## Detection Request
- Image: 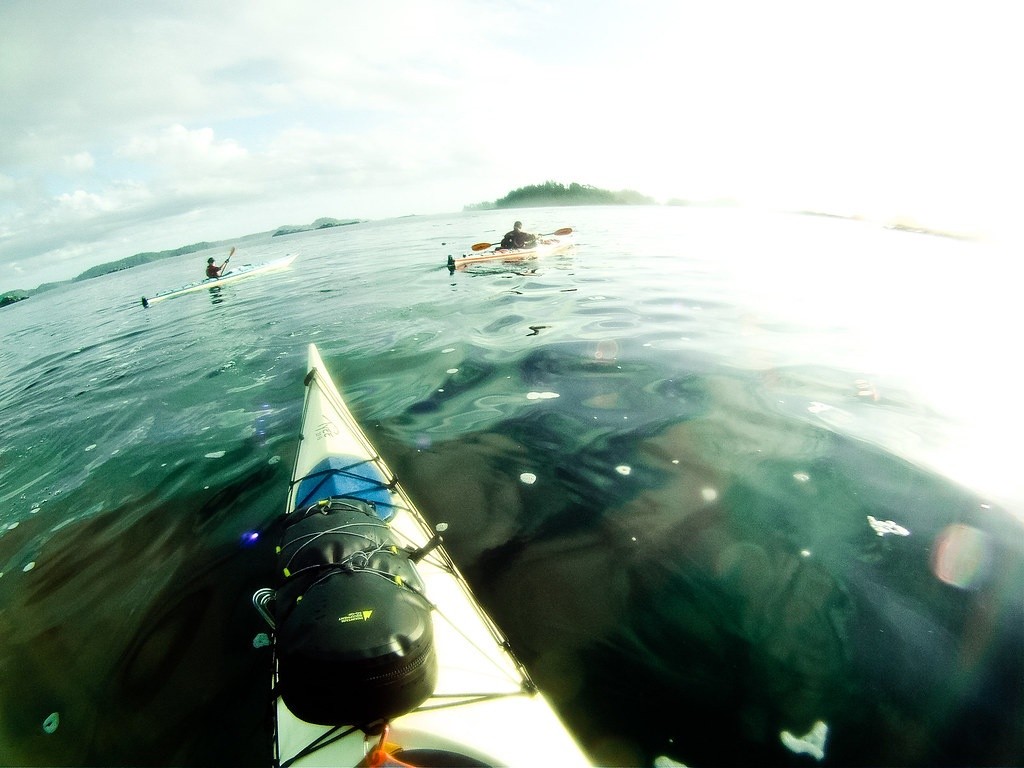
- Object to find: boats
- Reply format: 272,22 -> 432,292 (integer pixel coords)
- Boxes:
251,340 -> 604,768
140,251 -> 303,307
445,232 -> 570,272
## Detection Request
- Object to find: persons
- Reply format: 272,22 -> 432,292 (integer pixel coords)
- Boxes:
206,257 -> 228,278
502,222 -> 540,249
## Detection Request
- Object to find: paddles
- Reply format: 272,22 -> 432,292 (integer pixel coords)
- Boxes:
220,246 -> 235,276
471,227 -> 572,251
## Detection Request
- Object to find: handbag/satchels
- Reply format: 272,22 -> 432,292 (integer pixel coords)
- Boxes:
269,494 -> 437,728
